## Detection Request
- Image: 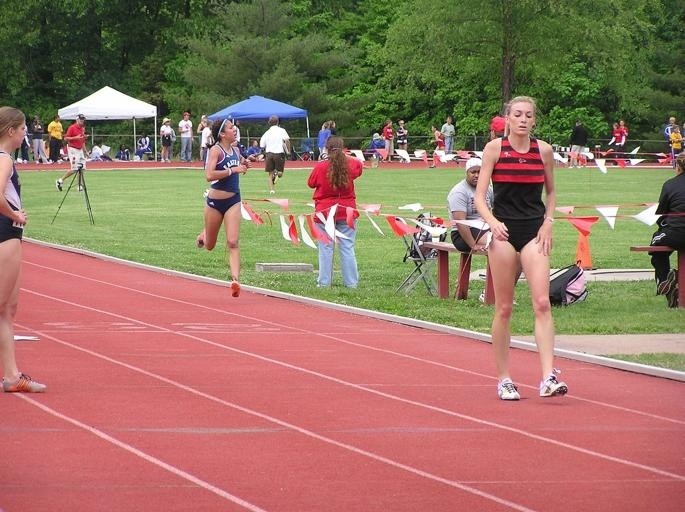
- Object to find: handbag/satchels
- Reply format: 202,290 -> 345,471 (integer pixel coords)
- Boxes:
162,135 -> 171,146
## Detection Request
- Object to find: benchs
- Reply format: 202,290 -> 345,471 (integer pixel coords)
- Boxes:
628,237 -> 685,316
364,152 -> 400,162
141,150 -> 153,160
421,236 -> 521,306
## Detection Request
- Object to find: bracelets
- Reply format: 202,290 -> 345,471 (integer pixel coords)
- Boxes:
543,213 -> 554,222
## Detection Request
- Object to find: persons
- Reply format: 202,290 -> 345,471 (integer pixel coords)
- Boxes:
21,115 -> 64,164
55,115 -> 90,192
135,131 -> 153,162
608,121 -> 629,165
159,115 -> 213,163
430,116 -> 455,168
448,158 -> 522,305
318,121 -> 337,160
233,121 -> 265,162
569,121 -> 588,167
370,120 -> 408,162
0,107 -> 45,393
307,136 -> 363,289
664,116 -> 682,160
197,120 -> 251,297
490,112 -> 506,140
475,96 -> 569,401
648,152 -> 684,308
260,116 -> 291,194
91,140 -> 129,161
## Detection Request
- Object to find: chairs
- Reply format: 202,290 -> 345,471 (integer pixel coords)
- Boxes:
387,213 -> 446,297
292,139 -> 315,161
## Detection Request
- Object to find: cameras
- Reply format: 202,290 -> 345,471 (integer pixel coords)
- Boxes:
75,162 -> 84,169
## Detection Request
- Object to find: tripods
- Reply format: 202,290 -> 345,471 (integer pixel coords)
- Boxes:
50,169 -> 95,225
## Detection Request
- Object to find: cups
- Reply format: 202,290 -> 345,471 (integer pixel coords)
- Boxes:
432,235 -> 440,242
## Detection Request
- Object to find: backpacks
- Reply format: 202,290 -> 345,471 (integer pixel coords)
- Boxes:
404,212 -> 447,261
550,260 -> 588,309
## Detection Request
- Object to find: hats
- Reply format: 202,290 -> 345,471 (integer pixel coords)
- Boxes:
491,116 -> 505,133
162,117 -> 171,124
465,158 -> 484,173
185,112 -> 190,117
77,113 -> 86,120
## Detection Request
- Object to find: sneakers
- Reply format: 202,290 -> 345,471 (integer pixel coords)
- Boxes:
56,179 -> 64,191
196,233 -> 204,248
230,280 -> 241,297
657,269 -> 678,295
539,374 -> 568,396
666,286 -> 678,308
497,378 -> 521,400
3,372 -> 46,393
